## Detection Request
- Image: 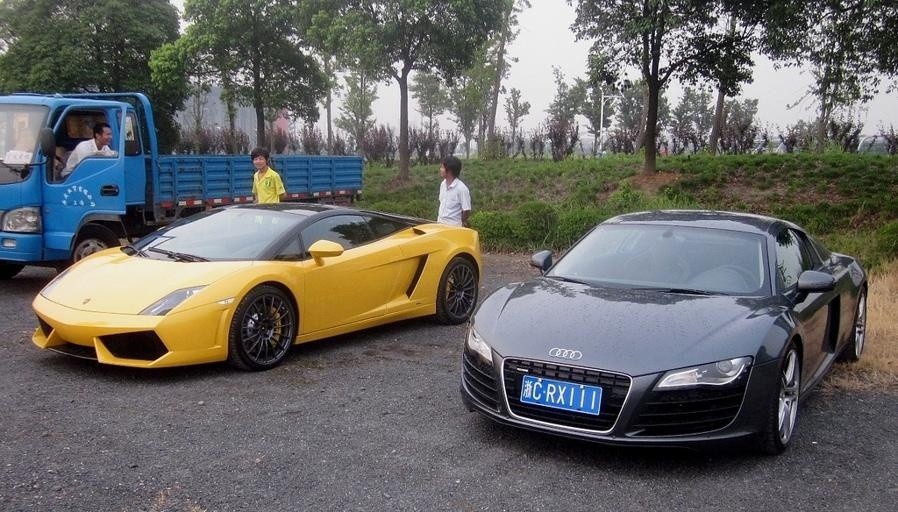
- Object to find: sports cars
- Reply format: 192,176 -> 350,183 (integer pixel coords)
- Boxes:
460,205 -> 870,461
30,201 -> 485,377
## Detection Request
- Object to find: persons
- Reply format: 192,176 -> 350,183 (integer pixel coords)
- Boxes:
436,156 -> 471,228
251,146 -> 286,203
60,123 -> 120,181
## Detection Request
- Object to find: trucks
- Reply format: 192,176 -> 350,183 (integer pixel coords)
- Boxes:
1,91 -> 366,286
